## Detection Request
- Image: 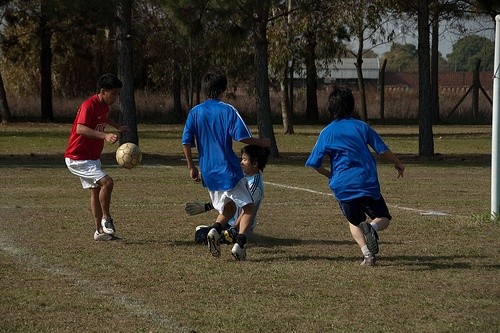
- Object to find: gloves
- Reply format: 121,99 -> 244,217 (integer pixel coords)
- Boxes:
185,200 -> 211,216
224,227 -> 236,242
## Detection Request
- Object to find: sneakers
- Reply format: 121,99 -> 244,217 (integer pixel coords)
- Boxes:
360,255 -> 376,267
207,228 -> 221,258
231,242 -> 248,261
359,221 -> 379,254
94,230 -> 114,241
101,217 -> 116,235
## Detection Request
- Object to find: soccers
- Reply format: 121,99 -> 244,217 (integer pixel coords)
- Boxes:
116,143 -> 142,169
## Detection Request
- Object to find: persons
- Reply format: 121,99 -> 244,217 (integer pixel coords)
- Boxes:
181,69 -> 271,262
185,143 -> 271,244
64,73 -> 131,240
304,85 -> 406,267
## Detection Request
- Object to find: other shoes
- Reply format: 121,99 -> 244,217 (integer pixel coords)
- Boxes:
196,225 -> 209,232
